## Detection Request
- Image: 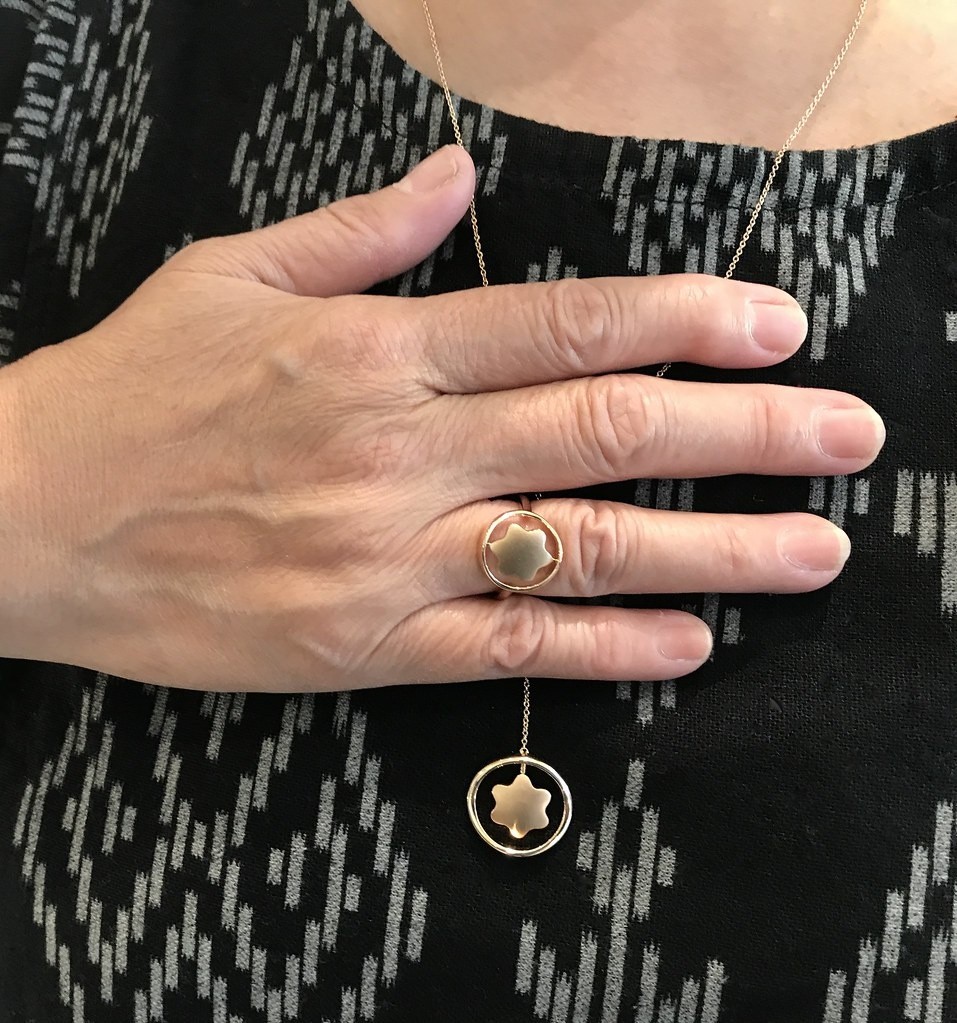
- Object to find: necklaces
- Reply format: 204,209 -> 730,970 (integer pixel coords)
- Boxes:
420,4 -> 869,858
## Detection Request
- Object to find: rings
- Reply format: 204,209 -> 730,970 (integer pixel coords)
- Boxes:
475,492 -> 568,601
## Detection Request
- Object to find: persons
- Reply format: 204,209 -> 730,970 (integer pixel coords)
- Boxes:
0,1 -> 957,1023
